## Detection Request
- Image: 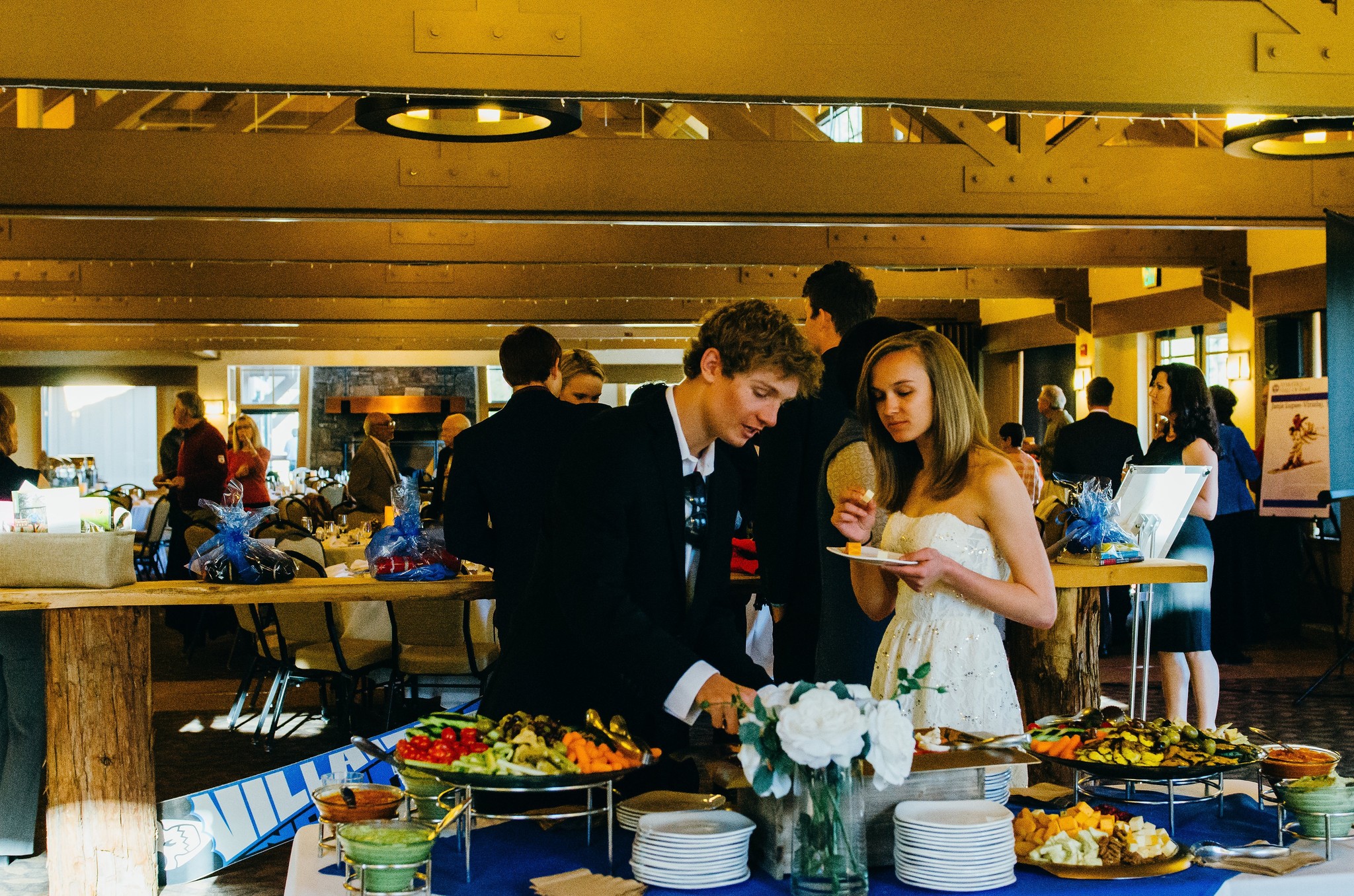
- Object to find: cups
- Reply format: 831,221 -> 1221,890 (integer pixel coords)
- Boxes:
265,467 -> 350,499
301,514 -> 372,546
321,771 -> 364,787
394,770 -> 458,820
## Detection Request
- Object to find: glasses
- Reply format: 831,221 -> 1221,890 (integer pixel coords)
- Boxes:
681,470 -> 713,550
237,425 -> 251,430
372,420 -> 396,427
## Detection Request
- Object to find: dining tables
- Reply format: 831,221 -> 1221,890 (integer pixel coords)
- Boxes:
268,490 -> 303,521
321,531 -> 372,568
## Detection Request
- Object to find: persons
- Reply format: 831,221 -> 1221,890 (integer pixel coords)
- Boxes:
444,326 -> 612,655
161,391 -> 271,656
754,259 -> 928,690
1208,385 -> 1260,665
1053,376 -> 1145,658
829,331 -> 1056,788
999,422 -> 1045,506
0,391 -> 43,502
1140,362 -> 1225,732
345,412 -> 403,514
1036,385 -> 1074,458
1282,413 -> 1318,470
474,299 -> 826,745
556,348 -> 607,404
422,413 -> 471,520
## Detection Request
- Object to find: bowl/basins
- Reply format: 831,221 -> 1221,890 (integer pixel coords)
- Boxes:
1276,778 -> 1354,838
1258,742 -> 1342,798
402,759 -> 659,812
312,782 -> 408,838
335,820 -> 439,894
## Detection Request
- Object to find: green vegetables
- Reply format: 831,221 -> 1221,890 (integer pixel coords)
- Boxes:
405,732 -> 549,774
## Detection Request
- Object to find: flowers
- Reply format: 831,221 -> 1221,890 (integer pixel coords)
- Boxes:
702,660 -> 949,894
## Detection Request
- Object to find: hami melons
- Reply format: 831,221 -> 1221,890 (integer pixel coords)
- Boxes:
1013,802 -> 1125,854
845,542 -> 862,556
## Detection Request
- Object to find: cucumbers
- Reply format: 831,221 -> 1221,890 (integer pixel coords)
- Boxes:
1026,727 -> 1086,741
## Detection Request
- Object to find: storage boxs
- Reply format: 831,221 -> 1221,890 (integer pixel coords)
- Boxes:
2,529 -> 137,590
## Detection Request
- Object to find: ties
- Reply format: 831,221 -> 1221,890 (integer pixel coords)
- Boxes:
177,440 -> 184,476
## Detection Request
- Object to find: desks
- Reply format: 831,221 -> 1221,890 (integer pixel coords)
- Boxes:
1052,557 -> 1209,719
0,572 -> 496,896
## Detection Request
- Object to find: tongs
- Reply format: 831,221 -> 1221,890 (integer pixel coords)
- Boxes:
1035,705 -> 1128,728
1190,839 -> 1292,863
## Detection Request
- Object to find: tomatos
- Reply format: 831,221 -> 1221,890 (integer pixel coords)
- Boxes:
1026,723 -> 1041,732
1058,724 -> 1068,729
396,727 -> 488,765
1100,722 -> 1113,728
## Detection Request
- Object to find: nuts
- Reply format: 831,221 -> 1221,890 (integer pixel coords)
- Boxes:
1095,823 -> 1169,864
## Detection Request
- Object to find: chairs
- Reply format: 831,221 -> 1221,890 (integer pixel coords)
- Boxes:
89,483 -> 501,734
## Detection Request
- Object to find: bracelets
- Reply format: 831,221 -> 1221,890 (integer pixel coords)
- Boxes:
771,603 -> 785,606
861,531 -> 873,546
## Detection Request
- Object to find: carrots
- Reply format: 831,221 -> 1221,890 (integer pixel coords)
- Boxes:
562,732 -> 661,773
1030,729 -> 1107,760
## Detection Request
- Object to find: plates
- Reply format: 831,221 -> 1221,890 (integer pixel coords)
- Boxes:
628,810 -> 759,889
825,546 -> 919,567
985,765 -> 1011,805
1022,744 -> 1268,780
1018,837 -> 1188,868
892,800 -> 1020,893
616,791 -> 726,832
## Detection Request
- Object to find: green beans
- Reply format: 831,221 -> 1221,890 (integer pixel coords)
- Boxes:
407,712 -> 498,740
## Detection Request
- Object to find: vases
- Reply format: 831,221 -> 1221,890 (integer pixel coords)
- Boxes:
793,759 -> 871,896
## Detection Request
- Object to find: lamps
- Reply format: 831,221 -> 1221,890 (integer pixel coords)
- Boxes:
355,95 -> 585,142
1222,116 -> 1354,162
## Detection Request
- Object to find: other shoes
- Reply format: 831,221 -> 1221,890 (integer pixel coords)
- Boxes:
1213,649 -> 1254,666
1110,646 -> 1131,655
1099,645 -> 1109,655
